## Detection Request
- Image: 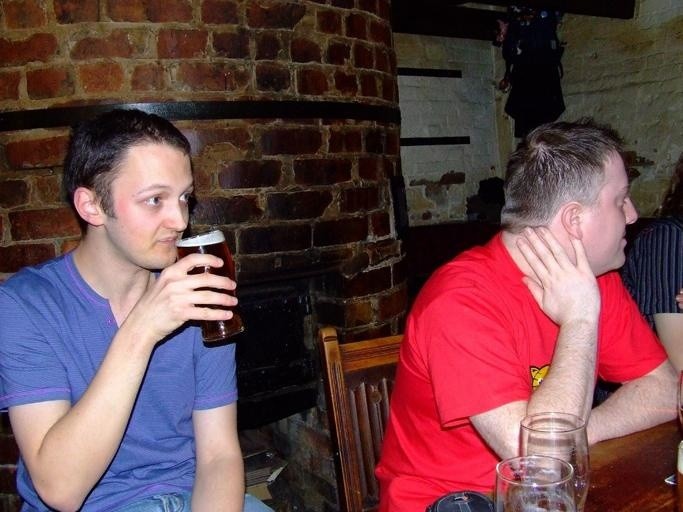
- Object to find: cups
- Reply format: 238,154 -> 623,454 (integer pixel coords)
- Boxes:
173,226 -> 245,345
494,456 -> 576,512
519,410 -> 590,511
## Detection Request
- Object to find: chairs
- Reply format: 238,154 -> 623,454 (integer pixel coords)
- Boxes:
312,318 -> 407,512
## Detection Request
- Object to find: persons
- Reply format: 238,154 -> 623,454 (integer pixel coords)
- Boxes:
0,109 -> 273,512
377,120 -> 683,511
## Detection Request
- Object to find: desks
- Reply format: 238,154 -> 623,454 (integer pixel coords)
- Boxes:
506,416 -> 683,511
